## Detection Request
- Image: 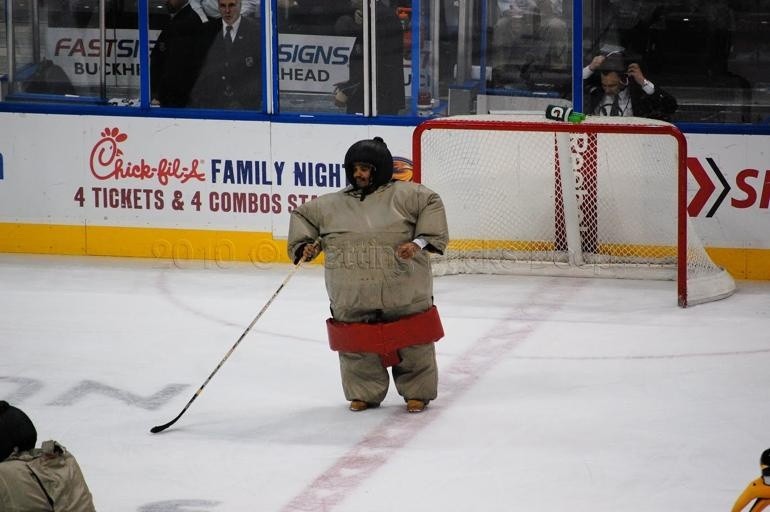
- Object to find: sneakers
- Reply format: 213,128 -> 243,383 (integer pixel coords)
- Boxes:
407,399 -> 426,411
349,399 -> 378,411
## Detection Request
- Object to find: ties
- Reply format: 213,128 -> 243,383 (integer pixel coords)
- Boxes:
224,25 -> 233,56
609,94 -> 619,116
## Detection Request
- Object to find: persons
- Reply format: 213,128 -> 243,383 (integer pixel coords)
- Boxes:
0,400 -> 95,512
287,137 -> 450,413
0,1 -> 677,120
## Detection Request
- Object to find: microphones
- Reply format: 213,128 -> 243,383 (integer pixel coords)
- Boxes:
619,74 -> 633,91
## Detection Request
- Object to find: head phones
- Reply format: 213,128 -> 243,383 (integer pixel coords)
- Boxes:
602,50 -> 632,80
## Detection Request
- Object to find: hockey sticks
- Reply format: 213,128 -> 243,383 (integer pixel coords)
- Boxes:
151,236 -> 322,434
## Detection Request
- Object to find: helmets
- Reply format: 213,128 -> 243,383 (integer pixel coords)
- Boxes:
0,400 -> 37,463
344,137 -> 397,191
